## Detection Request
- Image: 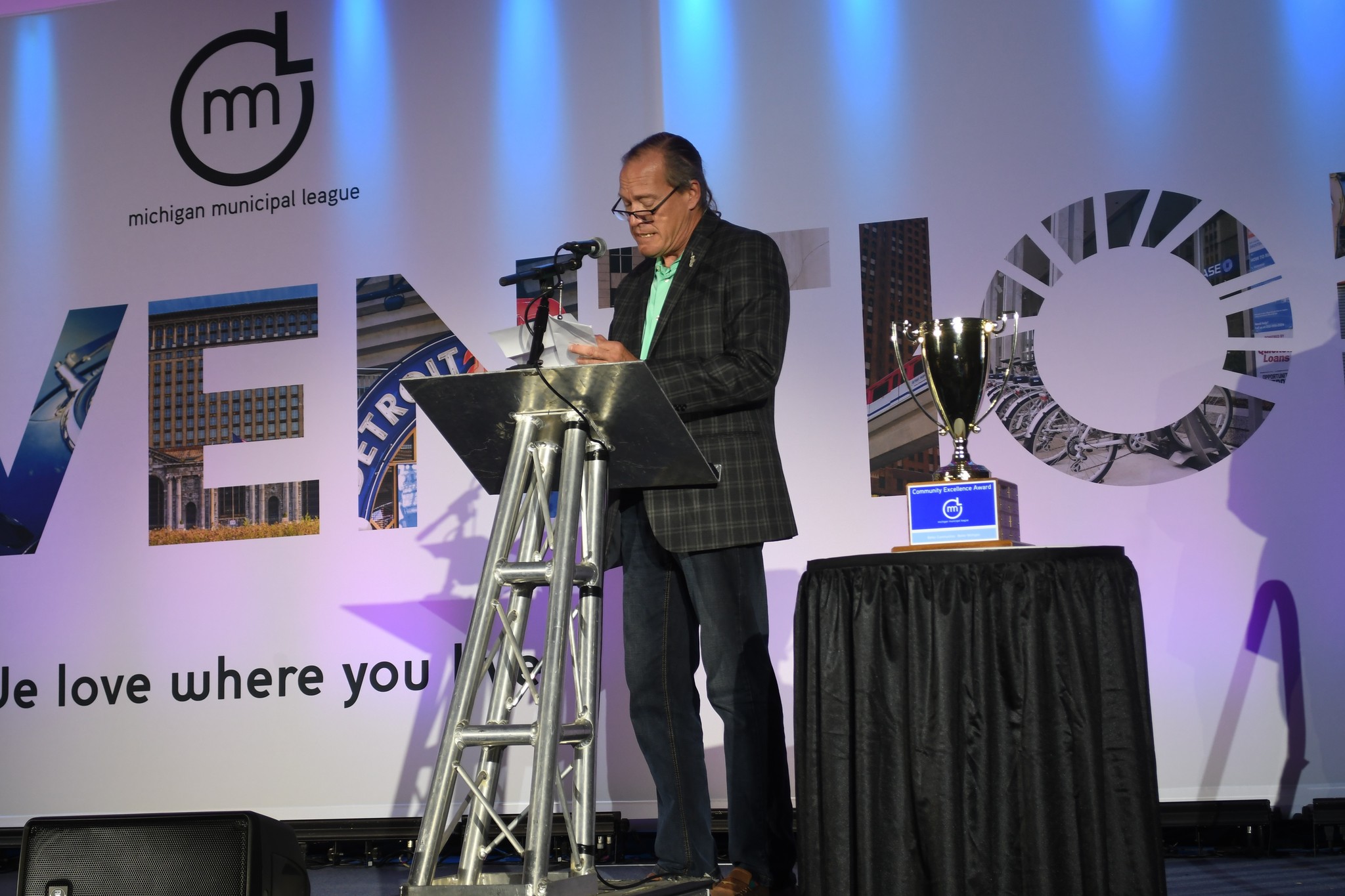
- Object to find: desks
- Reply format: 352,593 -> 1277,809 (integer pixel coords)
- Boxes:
793,543 -> 1168,896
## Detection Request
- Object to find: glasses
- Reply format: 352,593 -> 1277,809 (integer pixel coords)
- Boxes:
611,179 -> 689,222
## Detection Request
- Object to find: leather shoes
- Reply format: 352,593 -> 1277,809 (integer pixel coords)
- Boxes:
708,868 -> 797,896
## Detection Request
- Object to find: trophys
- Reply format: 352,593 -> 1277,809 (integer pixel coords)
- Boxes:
890,308 -> 1020,547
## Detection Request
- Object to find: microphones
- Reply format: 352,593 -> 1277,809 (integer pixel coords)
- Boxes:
559,237 -> 608,259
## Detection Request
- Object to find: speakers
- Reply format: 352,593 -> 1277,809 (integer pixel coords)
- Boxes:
13,812 -> 313,896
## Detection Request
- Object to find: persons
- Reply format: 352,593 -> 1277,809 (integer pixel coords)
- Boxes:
568,131 -> 799,896
